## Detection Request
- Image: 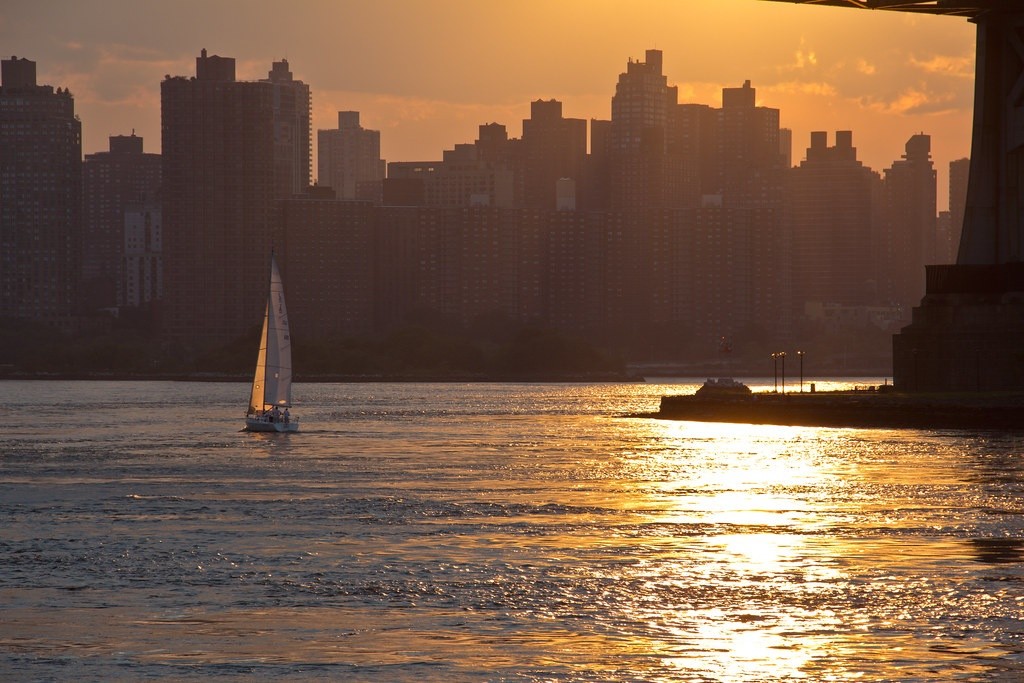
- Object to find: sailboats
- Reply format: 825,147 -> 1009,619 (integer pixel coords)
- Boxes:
245,247 -> 299,432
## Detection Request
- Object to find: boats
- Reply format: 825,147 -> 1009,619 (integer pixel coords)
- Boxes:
695,377 -> 752,396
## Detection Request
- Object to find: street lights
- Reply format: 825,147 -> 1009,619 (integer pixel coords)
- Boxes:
779,351 -> 787,394
771,352 -> 780,393
797,350 -> 806,393
